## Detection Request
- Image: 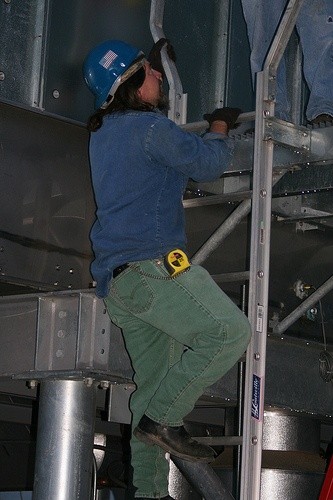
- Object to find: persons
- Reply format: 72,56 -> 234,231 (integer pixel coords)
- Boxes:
240,0 -> 333,131
82,40 -> 254,499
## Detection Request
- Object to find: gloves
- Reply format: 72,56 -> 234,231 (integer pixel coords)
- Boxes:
149,38 -> 176,75
204,106 -> 242,130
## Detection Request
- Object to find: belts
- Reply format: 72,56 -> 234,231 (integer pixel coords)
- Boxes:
111,262 -> 129,279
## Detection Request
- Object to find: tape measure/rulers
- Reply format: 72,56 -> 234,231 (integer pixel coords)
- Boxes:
164,247 -> 193,277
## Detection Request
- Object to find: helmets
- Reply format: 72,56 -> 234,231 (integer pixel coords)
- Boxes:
84,37 -> 147,109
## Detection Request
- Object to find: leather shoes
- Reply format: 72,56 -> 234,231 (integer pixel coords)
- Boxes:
133,414 -> 219,465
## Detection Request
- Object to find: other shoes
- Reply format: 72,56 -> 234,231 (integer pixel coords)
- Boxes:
307,115 -> 332,128
